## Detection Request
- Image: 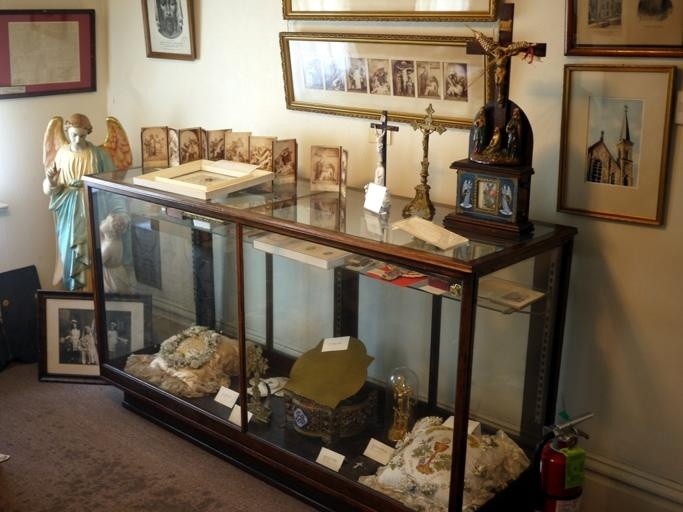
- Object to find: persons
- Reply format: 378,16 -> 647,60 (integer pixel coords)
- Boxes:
301,59 -> 468,101
459,180 -> 512,217
155,0 -> 179,36
100,213 -> 133,295
42,113 -> 133,292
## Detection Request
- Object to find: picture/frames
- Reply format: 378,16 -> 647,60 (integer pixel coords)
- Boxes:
0,8 -> 97,101
275,29 -> 495,132
32,285 -> 154,387
139,0 -> 200,63
278,0 -> 501,25
562,1 -> 683,61
551,62 -> 679,231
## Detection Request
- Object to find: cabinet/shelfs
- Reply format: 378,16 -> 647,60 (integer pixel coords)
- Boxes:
70,156 -> 584,512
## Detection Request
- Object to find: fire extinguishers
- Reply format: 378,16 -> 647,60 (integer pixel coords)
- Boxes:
534,412 -> 595,512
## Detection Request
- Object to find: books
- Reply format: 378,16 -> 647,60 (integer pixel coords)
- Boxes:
252,234 -> 350,270
165,206 -> 227,232
479,276 -> 544,312
